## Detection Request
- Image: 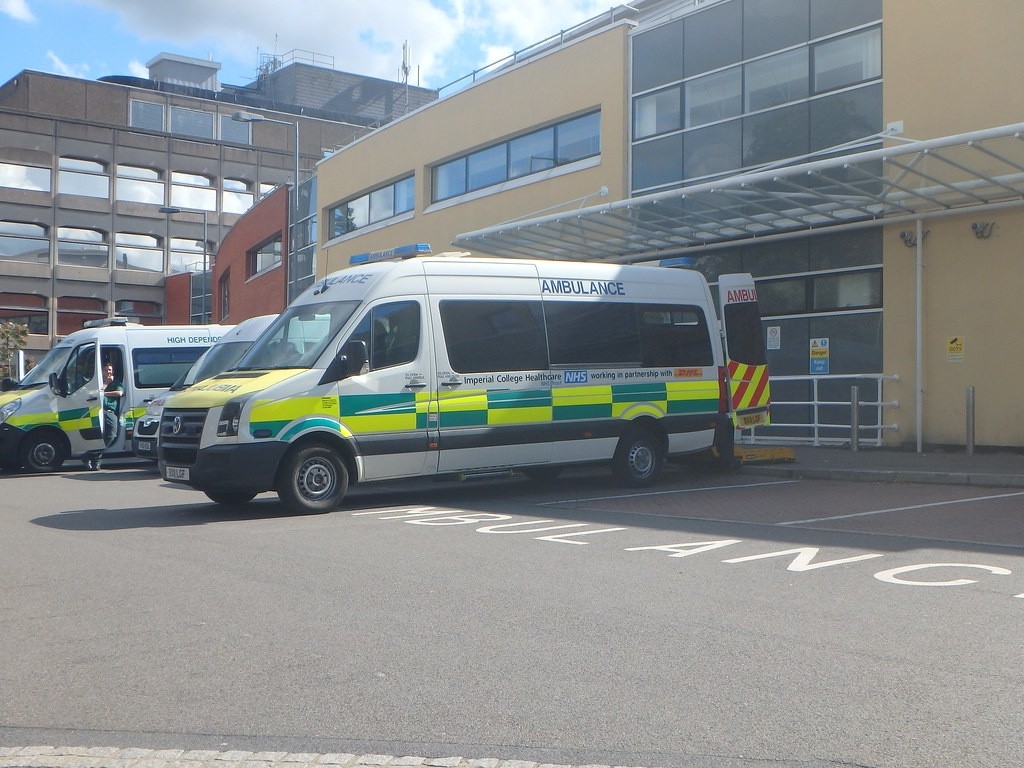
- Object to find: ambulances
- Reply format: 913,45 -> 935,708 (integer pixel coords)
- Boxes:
0,315 -> 237,472
157,243 -> 772,515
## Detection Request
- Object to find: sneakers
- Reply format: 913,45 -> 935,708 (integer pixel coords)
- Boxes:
83,459 -> 101,471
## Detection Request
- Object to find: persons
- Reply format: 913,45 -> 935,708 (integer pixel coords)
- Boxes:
83,362 -> 125,470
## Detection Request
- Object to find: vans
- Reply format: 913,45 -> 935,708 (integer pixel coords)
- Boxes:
131,314 -> 330,460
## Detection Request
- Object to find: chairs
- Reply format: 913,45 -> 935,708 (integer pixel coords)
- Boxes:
80,316 -> 419,394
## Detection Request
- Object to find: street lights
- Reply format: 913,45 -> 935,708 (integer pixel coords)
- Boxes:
158,207 -> 207,325
232,111 -> 299,301
